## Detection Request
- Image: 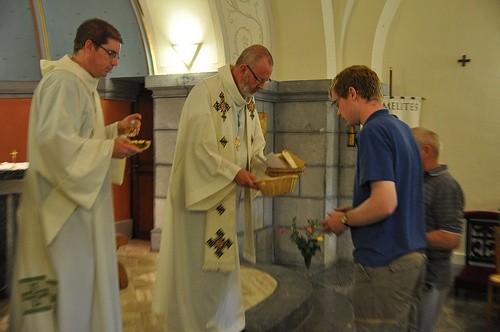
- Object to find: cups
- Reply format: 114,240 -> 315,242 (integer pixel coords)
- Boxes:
126,120 -> 139,138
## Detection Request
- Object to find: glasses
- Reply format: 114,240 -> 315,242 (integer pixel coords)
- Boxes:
92,41 -> 120,60
246,64 -> 272,84
330,96 -> 341,109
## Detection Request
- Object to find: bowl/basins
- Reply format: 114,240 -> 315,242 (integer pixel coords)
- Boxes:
130,140 -> 152,151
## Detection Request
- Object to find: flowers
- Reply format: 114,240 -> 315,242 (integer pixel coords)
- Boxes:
279,215 -> 324,272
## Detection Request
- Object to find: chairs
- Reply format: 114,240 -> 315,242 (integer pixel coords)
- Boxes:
486,226 -> 500,332
453,210 -> 500,299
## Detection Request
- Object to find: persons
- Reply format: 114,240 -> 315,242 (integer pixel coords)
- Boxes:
148,41 -> 275,331
2,16 -> 144,332
410,124 -> 468,332
320,64 -> 431,332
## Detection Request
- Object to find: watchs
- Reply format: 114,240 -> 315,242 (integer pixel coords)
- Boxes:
341,211 -> 351,228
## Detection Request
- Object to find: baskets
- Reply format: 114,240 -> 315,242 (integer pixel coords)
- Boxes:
266,167 -> 306,178
253,174 -> 299,196
